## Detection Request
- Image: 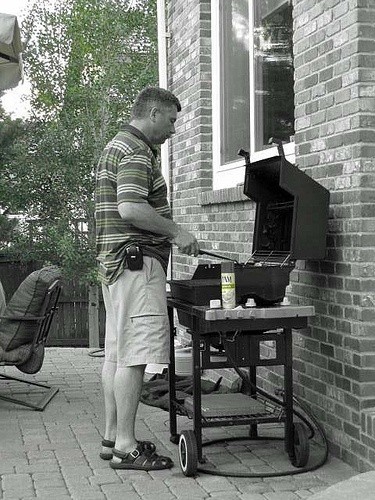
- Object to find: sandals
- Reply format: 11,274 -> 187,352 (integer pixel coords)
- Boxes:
111,447 -> 174,470
101,438 -> 156,460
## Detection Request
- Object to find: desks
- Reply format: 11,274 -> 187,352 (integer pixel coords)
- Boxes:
167,291 -> 316,478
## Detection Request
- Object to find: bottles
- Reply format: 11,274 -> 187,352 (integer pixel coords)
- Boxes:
221,262 -> 235,309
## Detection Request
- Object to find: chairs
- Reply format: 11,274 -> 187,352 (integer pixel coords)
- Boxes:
0,265 -> 64,413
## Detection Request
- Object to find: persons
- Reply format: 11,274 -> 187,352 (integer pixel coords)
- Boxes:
94,86 -> 200,470
